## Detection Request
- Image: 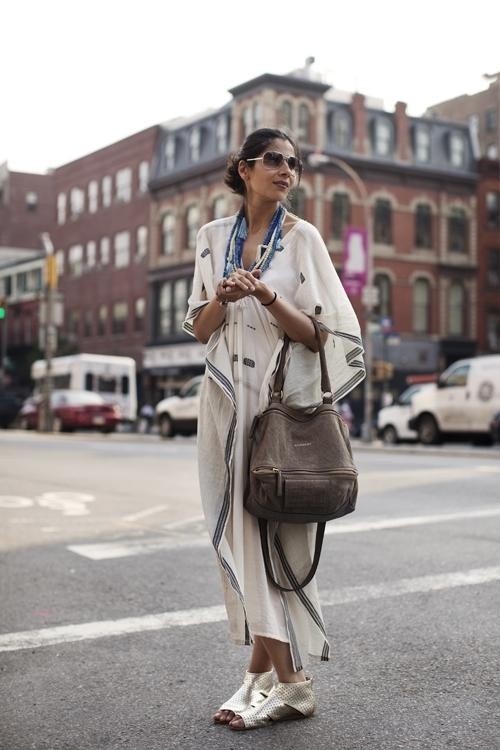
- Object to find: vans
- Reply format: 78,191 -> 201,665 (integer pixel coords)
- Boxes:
30,352 -> 138,424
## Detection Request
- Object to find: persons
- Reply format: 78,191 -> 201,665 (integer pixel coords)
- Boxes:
181,128 -> 365,731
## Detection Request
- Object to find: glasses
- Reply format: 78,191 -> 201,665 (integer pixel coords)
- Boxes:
247,151 -> 302,175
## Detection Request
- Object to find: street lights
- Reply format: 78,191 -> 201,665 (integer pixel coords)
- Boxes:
307,151 -> 376,443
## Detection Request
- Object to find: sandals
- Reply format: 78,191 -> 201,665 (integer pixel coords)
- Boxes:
213,668 -> 317,730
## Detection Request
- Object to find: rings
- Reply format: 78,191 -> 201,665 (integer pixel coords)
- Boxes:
220,297 -> 230,308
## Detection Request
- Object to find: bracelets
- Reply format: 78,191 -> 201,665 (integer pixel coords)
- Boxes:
260,292 -> 280,307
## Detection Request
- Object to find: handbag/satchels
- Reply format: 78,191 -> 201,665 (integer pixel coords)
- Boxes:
243,316 -> 358,523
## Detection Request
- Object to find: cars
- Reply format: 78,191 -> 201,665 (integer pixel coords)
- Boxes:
376,383 -> 438,444
407,352 -> 500,447
16,388 -> 123,433
155,373 -> 205,439
